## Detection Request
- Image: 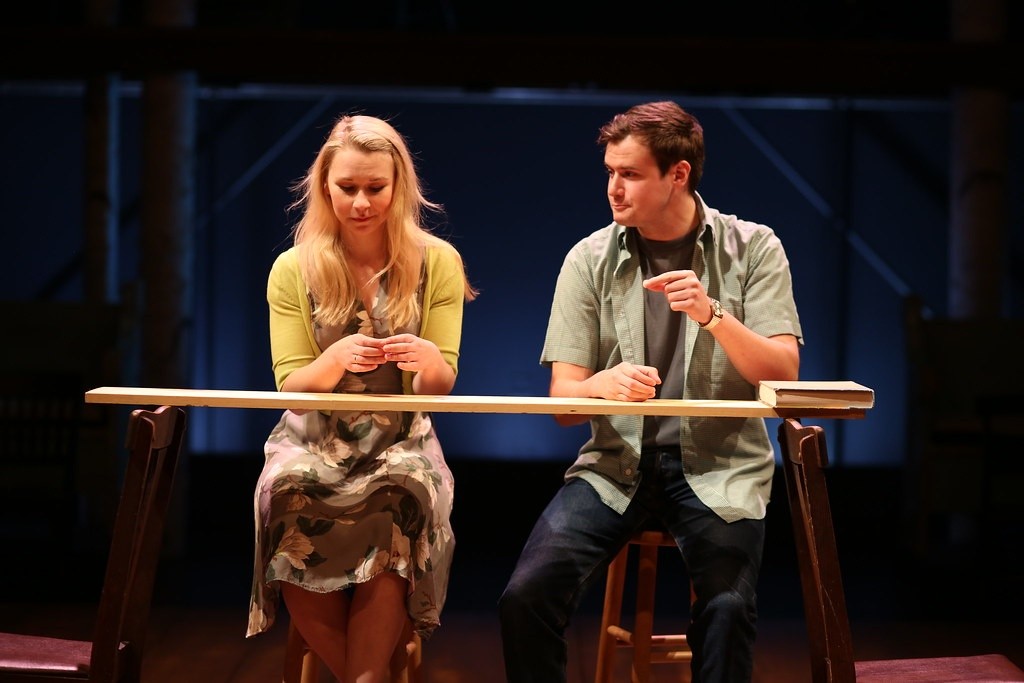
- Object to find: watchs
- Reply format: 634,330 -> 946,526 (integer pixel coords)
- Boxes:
697,298 -> 723,330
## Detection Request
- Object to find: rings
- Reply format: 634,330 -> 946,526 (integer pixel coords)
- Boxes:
355,355 -> 358,363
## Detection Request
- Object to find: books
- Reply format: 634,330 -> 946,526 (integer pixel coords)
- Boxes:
759,380 -> 875,409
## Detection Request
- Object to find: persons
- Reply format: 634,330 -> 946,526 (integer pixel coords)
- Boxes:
498,101 -> 805,683
246,115 -> 481,683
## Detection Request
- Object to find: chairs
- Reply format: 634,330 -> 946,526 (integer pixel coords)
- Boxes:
776,418 -> 1024,683
0,405 -> 187,683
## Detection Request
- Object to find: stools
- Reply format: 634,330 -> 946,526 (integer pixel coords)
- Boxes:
593,531 -> 701,683
283,614 -> 423,683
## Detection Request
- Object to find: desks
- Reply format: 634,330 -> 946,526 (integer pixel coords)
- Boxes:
83,386 -> 876,682
